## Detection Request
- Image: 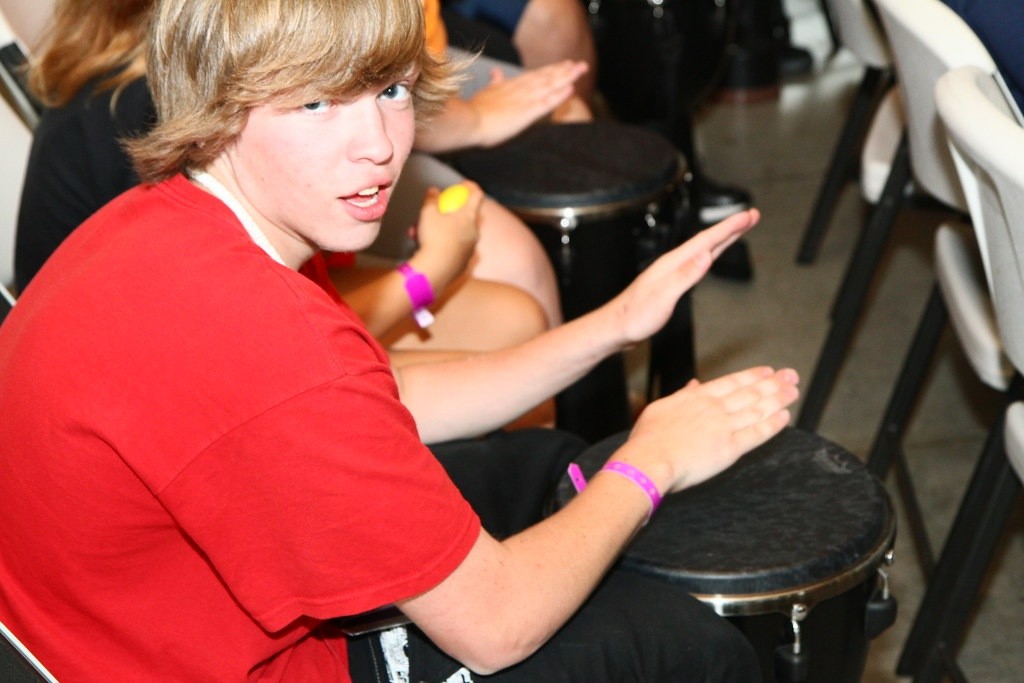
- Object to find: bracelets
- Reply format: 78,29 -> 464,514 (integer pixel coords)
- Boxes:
566,461 -> 662,526
396,262 -> 435,329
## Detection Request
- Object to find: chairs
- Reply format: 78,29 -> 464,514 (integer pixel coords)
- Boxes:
789,0 -> 1024,683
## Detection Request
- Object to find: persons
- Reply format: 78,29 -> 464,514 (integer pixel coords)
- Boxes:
411,0 -> 589,155
440,0 -> 752,223
0,0 -> 802,683
14,1 -> 560,429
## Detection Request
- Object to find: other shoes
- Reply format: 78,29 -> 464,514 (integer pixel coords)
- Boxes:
707,57 -> 777,103
693,176 -> 756,224
743,44 -> 813,102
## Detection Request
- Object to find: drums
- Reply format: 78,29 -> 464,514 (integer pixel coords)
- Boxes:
557,426 -> 899,682
451,119 -> 691,446
